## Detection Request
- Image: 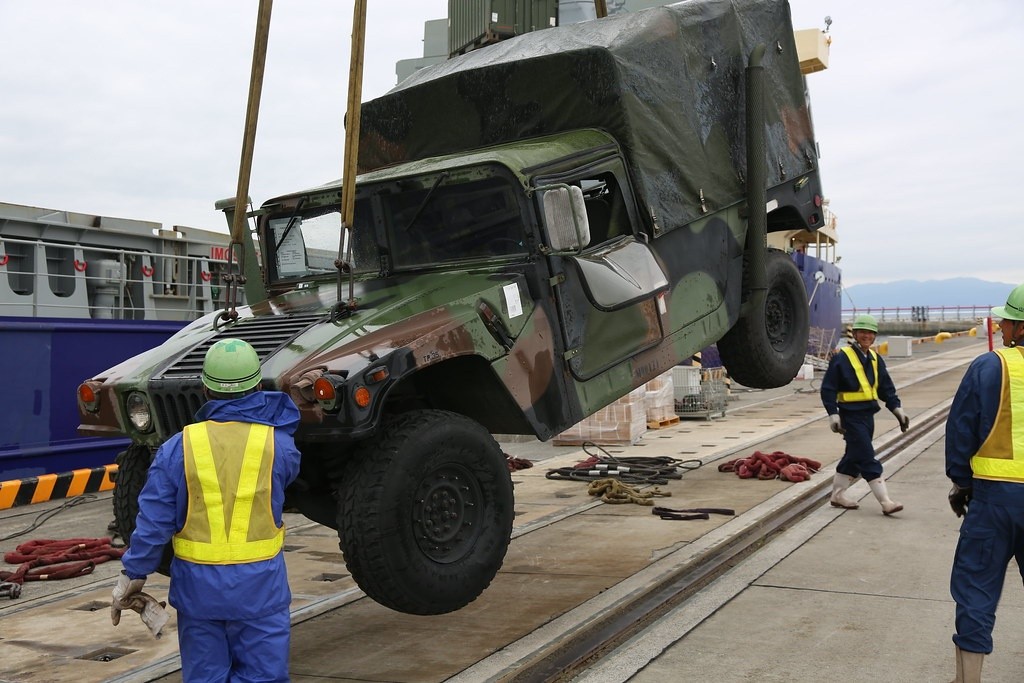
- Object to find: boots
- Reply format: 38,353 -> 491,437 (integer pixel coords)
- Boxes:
950,645 -> 985,683
868,477 -> 903,513
831,472 -> 859,509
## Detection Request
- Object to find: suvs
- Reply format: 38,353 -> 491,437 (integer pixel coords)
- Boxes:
76,0 -> 827,617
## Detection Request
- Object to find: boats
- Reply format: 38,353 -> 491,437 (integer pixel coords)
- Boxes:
0,187 -> 841,482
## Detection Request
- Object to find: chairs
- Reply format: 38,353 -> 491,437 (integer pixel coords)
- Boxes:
583,197 -> 610,250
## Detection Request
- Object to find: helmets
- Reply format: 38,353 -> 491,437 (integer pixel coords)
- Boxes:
201,338 -> 263,393
852,314 -> 879,334
991,284 -> 1024,320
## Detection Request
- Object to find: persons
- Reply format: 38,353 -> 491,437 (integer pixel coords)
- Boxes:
821,313 -> 909,515
946,286 -> 1024,683
112,339 -> 301,683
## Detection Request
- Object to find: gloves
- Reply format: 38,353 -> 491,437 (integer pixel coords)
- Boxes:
111,570 -> 170,640
947,483 -> 972,517
829,414 -> 846,434
892,408 -> 909,433
